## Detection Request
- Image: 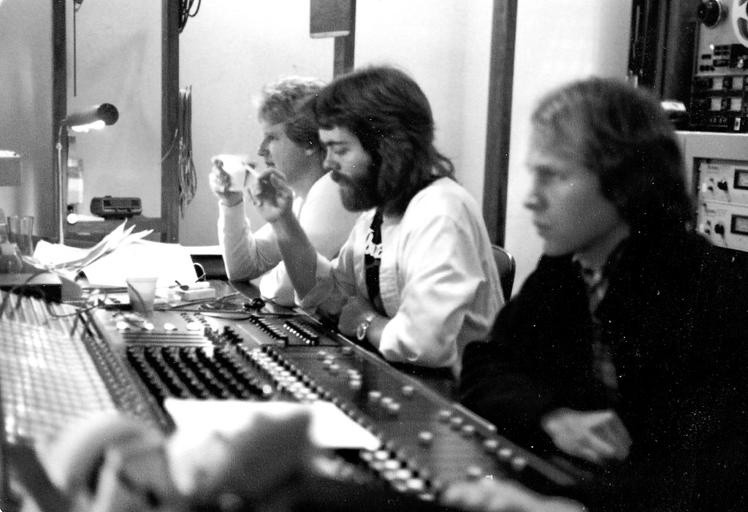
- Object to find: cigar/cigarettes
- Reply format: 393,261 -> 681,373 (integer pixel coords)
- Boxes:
245,164 -> 268,184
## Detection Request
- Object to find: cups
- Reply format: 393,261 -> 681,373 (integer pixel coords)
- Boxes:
127,278 -> 156,313
214,154 -> 250,193
8,216 -> 34,256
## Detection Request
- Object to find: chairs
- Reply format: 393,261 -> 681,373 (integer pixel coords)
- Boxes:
491,243 -> 515,301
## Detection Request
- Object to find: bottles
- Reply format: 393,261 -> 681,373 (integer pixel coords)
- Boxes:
67,136 -> 85,220
0,211 -> 23,275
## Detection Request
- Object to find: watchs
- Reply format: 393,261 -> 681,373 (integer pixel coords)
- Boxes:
356,312 -> 378,341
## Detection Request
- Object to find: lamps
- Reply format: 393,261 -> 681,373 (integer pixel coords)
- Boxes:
58,102 -> 119,243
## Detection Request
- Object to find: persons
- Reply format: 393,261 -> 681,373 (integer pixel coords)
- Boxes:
455,76 -> 748,512
248,65 -> 506,382
209,75 -> 363,309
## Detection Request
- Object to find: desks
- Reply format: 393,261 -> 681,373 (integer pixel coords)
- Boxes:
1,229 -> 651,512
